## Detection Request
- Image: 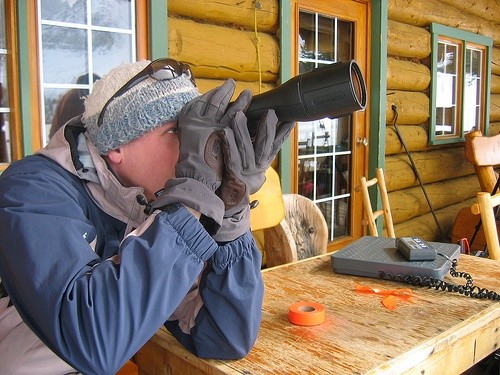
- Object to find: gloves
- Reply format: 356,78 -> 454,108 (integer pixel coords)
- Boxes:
212,110 -> 296,243
148,78 -> 253,237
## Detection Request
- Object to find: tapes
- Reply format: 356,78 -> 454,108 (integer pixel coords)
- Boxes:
288,300 -> 326,327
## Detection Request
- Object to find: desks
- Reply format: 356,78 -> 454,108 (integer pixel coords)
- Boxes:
133,250 -> 500,375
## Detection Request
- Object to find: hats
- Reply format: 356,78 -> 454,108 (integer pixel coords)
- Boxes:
79,59 -> 202,156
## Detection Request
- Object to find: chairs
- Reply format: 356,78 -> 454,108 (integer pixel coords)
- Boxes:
451,130 -> 500,254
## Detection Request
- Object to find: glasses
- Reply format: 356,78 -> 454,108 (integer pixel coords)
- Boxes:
97,57 -> 197,128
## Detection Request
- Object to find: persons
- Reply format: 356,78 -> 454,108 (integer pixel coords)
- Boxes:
0,58 -> 296,375
49,73 -> 101,140
